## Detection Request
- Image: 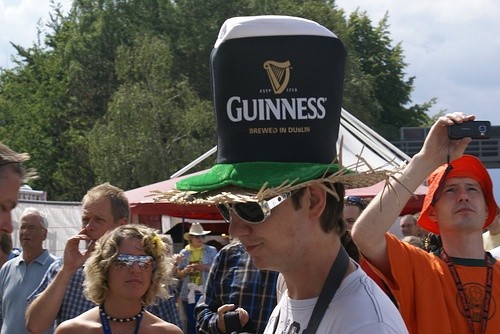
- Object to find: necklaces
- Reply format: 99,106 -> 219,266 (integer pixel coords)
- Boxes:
99,302 -> 146,323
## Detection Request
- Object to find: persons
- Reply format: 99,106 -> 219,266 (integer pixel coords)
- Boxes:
0,15 -> 500,334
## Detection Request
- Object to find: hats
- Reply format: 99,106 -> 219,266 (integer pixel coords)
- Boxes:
416,154 -> 498,236
145,134 -> 418,213
173,15 -> 355,192
183,222 -> 211,240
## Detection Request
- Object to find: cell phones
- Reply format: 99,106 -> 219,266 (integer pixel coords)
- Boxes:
448,121 -> 491,140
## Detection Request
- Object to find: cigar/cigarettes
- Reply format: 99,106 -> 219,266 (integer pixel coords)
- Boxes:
88,240 -> 93,247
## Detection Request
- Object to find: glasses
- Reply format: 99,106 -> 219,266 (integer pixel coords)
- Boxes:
191,236 -> 205,239
215,188 -> 301,224
111,253 -> 157,271
344,196 -> 366,209
